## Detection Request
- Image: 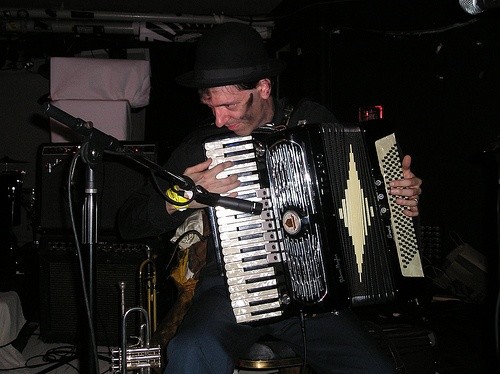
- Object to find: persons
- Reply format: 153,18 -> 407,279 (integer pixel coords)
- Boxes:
126,42 -> 424,374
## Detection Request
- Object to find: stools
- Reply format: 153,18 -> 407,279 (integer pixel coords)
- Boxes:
233,331 -> 311,374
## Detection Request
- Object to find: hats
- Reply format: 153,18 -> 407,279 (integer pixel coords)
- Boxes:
191,21 -> 272,89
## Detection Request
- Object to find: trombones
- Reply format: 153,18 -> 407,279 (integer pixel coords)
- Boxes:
138,252 -> 158,348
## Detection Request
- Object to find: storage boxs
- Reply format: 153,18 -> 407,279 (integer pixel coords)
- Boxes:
50,56 -> 152,111
49,98 -> 134,146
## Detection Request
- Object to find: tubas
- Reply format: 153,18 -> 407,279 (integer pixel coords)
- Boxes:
112,282 -> 162,374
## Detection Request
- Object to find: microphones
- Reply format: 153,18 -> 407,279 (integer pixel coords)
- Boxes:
187,188 -> 263,213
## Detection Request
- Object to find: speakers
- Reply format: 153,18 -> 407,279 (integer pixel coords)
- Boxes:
34,235 -> 152,350
